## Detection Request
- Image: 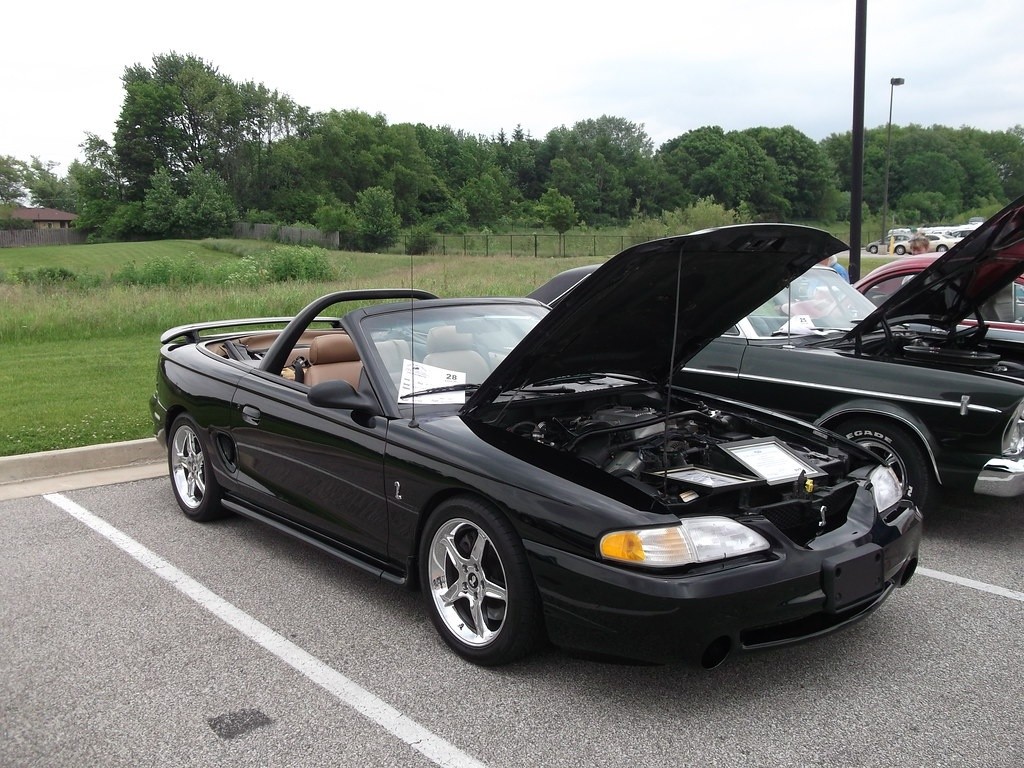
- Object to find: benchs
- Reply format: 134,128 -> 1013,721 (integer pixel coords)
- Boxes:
283,340 -> 414,389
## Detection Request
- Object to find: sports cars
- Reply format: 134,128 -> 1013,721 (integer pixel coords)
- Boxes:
148,220 -> 926,669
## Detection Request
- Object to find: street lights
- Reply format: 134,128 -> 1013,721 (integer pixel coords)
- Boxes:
879,77 -> 905,245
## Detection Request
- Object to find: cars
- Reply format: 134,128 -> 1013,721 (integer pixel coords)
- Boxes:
520,193 -> 1023,522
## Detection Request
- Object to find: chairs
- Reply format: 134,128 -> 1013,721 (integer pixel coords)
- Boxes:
305,334 -> 363,391
422,326 -> 490,385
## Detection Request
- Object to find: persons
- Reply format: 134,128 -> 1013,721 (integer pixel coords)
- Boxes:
901,232 -> 929,285
806,254 -> 849,316
